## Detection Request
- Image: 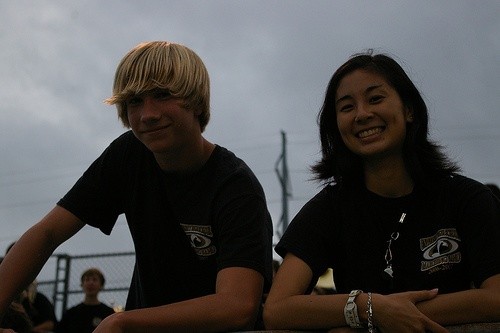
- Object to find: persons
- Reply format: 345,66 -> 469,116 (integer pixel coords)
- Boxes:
261,48 -> 500,333
58,268 -> 116,333
0,241 -> 56,333
0,40 -> 273,333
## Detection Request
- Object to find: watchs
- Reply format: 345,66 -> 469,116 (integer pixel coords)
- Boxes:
344,289 -> 362,330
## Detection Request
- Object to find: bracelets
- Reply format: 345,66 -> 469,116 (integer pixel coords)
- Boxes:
366,292 -> 374,333
